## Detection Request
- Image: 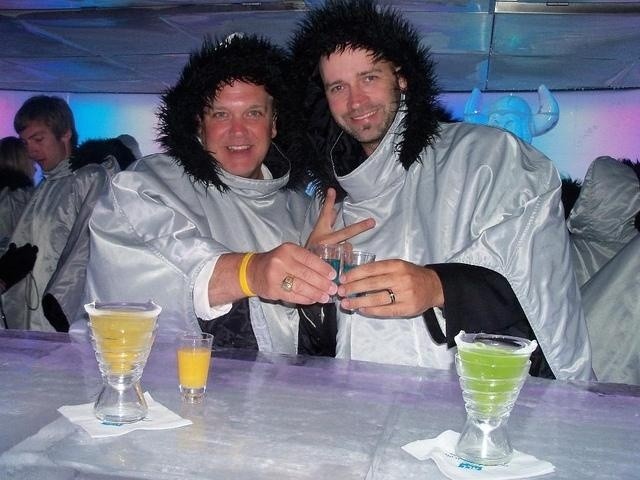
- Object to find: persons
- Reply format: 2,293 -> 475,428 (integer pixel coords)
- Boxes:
117,132 -> 143,162
0,136 -> 37,330
553,156 -> 639,388
289,0 -> 599,383
0,93 -> 124,337
75,34 -> 339,359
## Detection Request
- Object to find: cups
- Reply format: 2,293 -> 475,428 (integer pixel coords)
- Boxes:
176,330 -> 214,404
312,241 -> 341,305
340,246 -> 374,312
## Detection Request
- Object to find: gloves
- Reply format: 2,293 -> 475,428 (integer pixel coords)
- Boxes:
1,242 -> 38,291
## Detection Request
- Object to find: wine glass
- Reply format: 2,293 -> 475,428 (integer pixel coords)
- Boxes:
82,299 -> 164,424
451,330 -> 535,465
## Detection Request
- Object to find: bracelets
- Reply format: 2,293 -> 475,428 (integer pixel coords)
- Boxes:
238,251 -> 259,297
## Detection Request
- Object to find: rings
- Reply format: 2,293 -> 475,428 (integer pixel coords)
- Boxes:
385,288 -> 396,305
280,274 -> 295,293
